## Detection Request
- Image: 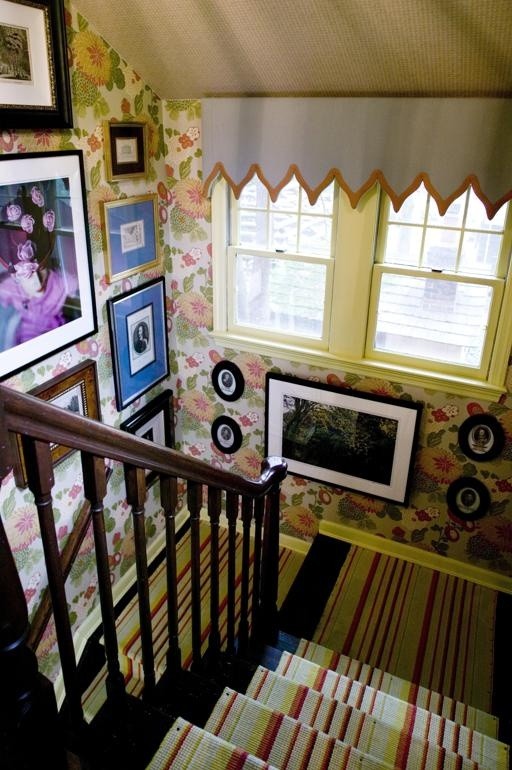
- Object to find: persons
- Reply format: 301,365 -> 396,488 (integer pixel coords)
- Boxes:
134,325 -> 149,354
0,258 -> 80,345
222,374 -> 232,387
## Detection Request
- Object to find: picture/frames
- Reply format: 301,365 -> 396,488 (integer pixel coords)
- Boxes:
212,360 -> 244,401
264,371 -> 423,508
458,413 -> 504,462
211,415 -> 242,454
446,476 -> 491,520
